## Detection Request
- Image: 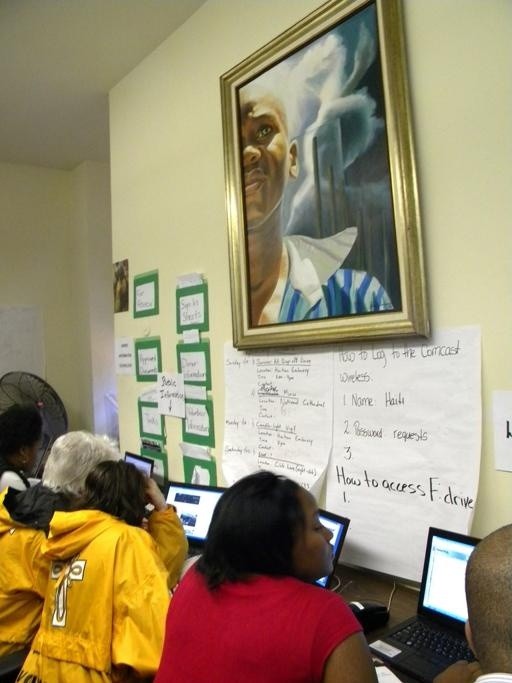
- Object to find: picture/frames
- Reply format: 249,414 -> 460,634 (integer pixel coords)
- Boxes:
219,0 -> 430,348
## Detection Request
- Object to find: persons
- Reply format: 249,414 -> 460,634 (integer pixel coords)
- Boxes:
0,429 -> 123,683
238,84 -> 394,326
0,402 -> 44,493
151,469 -> 378,683
14,459 -> 191,683
432,521 -> 512,683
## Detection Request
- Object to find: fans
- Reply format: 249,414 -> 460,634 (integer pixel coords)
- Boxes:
1,371 -> 68,477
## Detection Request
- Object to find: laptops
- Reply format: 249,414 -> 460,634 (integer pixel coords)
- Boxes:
315,508 -> 351,591
367,527 -> 484,683
163,480 -> 230,559
124,451 -> 154,483
